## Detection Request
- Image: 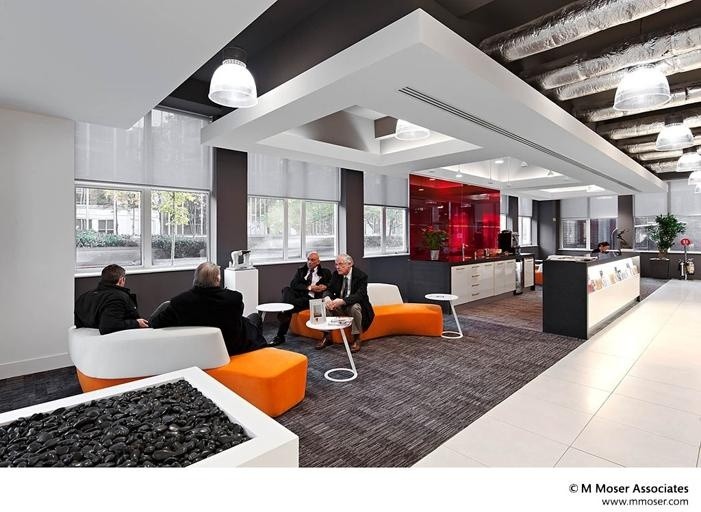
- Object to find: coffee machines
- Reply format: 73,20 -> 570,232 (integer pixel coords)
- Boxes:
498,230 -> 519,255
468,218 -> 485,242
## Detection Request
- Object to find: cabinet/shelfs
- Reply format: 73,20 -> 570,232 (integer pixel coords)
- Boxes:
519,252 -> 538,295
409,255 -> 516,310
541,251 -> 645,339
224,264 -> 261,319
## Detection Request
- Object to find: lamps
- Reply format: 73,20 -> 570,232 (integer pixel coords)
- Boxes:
656,114 -> 696,152
687,173 -> 701,187
393,113 -> 430,141
206,44 -> 260,110
672,145 -> 701,171
450,155 -> 613,187
610,61 -> 675,110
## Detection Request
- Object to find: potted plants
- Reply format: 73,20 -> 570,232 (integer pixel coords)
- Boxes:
646,208 -> 688,257
423,225 -> 450,260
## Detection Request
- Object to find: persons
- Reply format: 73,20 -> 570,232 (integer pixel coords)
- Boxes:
266,252 -> 332,345
151,263 -> 266,356
315,254 -> 374,353
592,242 -> 610,254
75,264 -> 171,335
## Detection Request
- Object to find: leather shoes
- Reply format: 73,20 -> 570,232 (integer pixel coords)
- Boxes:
315,336 -> 333,349
350,341 -> 360,352
267,337 -> 285,347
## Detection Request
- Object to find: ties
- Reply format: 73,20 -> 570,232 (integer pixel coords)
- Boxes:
307,269 -> 314,286
343,277 -> 348,298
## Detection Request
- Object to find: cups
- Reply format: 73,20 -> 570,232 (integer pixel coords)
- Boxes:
477,247 -> 503,256
584,253 -> 591,259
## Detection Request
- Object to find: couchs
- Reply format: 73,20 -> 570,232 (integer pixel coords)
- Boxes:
287,279 -> 445,346
63,323 -> 312,418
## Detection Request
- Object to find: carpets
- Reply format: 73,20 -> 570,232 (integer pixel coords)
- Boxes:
0,314 -> 592,468
453,271 -> 674,340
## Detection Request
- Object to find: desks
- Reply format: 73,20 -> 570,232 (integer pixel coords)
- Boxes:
425,292 -> 467,341
252,303 -> 296,314
648,257 -> 670,280
307,314 -> 360,382
